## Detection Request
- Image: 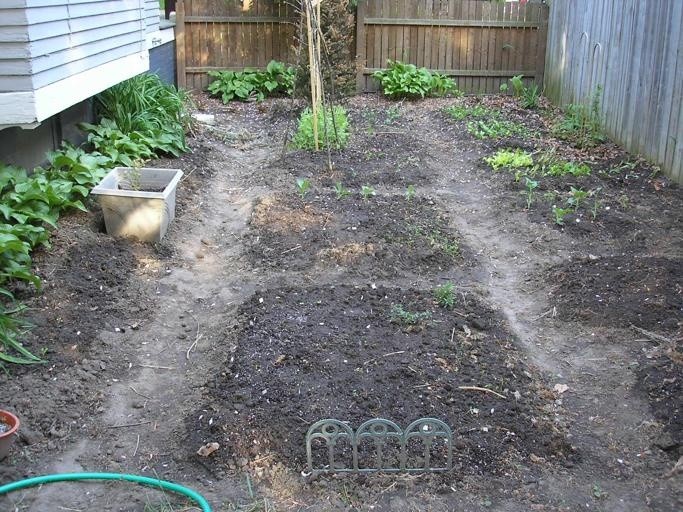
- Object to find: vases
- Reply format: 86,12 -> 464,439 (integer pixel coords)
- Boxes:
89,166 -> 184,244
0,410 -> 20,462
